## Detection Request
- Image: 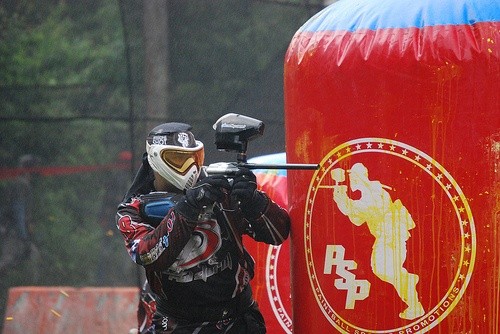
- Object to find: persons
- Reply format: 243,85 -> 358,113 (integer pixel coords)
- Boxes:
332,163 -> 429,320
115,123 -> 290,334
